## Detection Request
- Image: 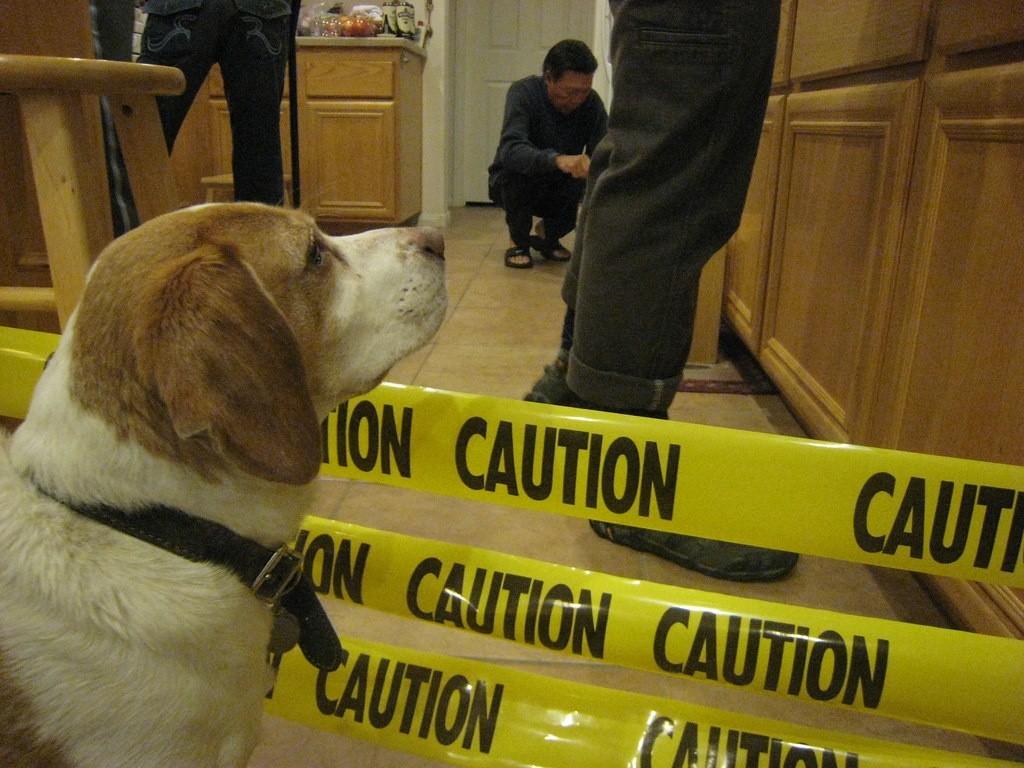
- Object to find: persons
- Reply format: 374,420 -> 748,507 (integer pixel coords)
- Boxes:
488,40 -> 608,269
523,1 -> 800,580
82,0 -> 290,245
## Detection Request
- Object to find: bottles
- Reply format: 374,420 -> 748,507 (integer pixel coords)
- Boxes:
416,21 -> 425,46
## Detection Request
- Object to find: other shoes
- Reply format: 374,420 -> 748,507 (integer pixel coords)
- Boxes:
589,511 -> 799,579
522,363 -> 576,406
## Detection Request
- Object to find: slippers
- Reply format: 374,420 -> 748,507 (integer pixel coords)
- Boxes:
528,234 -> 571,261
504,247 -> 533,268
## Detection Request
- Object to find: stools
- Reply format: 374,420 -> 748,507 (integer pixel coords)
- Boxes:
0,54 -> 186,338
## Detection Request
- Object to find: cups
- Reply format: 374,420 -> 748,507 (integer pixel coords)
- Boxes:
382,1 -> 416,39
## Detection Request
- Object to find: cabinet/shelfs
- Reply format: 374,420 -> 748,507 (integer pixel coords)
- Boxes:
723,0 -> 1023,467
169,46 -> 423,225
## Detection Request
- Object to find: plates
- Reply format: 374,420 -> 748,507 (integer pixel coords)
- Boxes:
376,33 -> 397,37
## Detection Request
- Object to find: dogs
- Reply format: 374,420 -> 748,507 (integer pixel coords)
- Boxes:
0,200 -> 451,768
